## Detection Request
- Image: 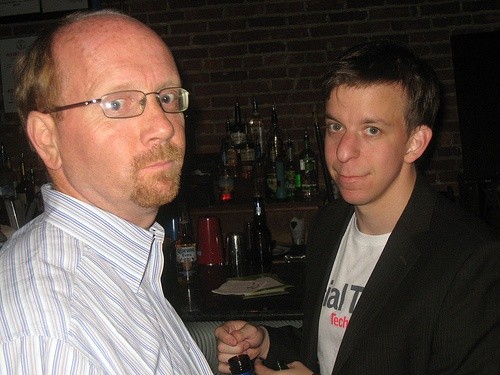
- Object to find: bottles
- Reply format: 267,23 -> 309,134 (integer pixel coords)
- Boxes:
267,136 -> 297,204
245,98 -> 283,162
217,103 -> 245,207
228,354 -> 255,375
297,124 -> 339,202
174,206 -> 198,280
250,196 -> 273,274
271,360 -> 288,371
0,145 -> 17,200
241,125 -> 267,206
17,153 -> 41,210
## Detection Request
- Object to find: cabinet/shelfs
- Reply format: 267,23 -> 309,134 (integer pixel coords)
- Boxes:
162,184 -> 325,323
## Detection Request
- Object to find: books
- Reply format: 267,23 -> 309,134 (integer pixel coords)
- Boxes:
224,274 -> 285,295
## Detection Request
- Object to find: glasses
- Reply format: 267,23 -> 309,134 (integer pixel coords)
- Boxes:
42,87 -> 189,119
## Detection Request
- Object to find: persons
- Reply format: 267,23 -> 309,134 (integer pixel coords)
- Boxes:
214,37 -> 500,375
0,9 -> 212,375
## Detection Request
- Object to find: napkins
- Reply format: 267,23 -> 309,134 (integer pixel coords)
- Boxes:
210,279 -> 264,297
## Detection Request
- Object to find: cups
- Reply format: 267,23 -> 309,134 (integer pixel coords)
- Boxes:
197,215 -> 224,266
226,232 -> 242,266
